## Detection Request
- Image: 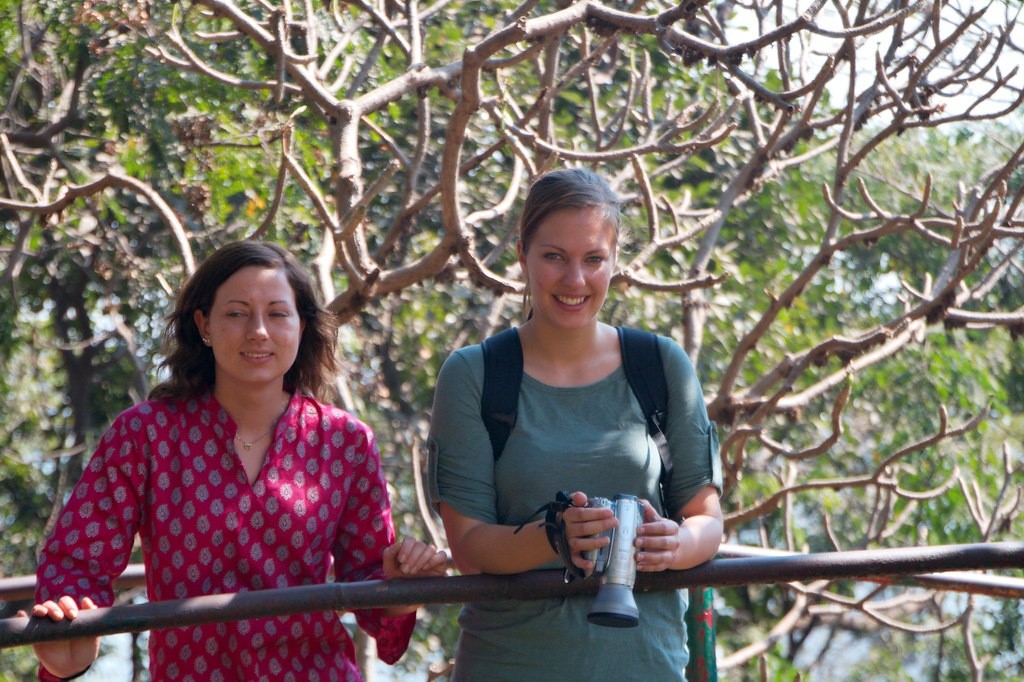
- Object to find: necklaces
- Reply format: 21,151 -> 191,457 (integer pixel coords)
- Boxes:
235,398 -> 284,451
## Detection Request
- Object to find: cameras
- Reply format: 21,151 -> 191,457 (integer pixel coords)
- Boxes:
577,493 -> 647,628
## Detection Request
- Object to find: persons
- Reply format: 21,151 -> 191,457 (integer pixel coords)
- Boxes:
16,241 -> 447,682
427,167 -> 725,682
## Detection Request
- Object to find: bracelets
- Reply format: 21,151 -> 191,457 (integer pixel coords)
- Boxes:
61,664 -> 92,681
